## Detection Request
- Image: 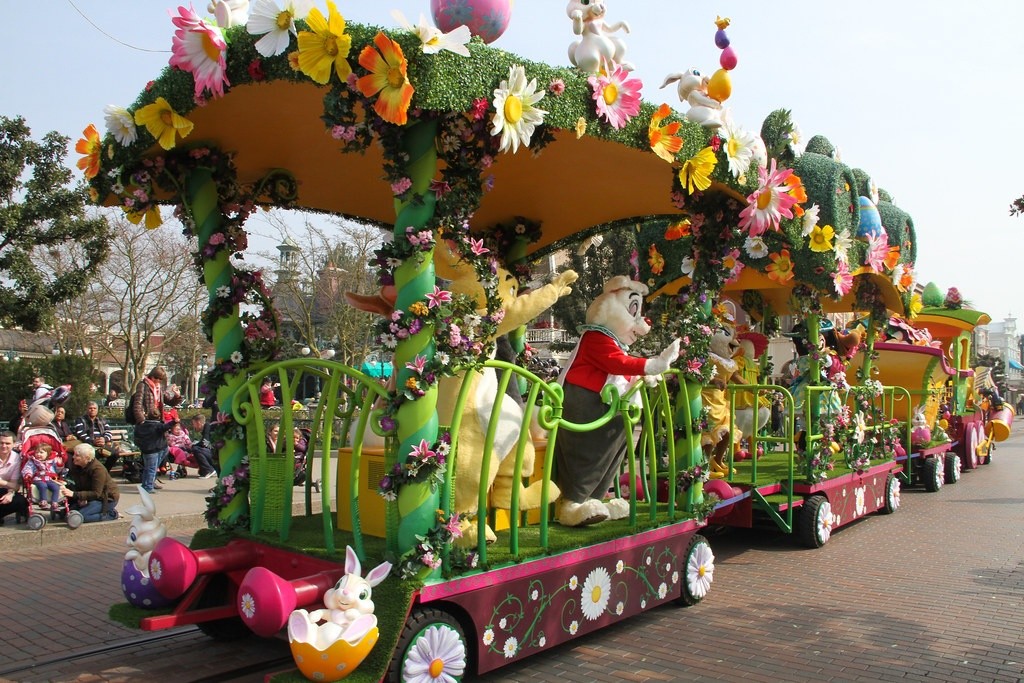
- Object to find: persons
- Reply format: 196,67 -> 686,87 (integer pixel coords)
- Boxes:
0,367 -> 307,527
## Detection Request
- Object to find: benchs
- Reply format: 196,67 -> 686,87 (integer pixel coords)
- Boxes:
105,429 -> 141,463
336,437 -> 550,539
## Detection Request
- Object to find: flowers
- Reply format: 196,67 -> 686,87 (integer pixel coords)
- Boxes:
76,1 -> 974,577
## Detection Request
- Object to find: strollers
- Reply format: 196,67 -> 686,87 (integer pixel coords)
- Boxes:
163,409 -> 199,480
293,428 -> 322,493
20,422 -> 84,531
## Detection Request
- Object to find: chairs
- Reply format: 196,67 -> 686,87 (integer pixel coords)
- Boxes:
101,396 -> 125,407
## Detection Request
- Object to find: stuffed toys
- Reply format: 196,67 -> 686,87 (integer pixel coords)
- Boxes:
783,317 -> 860,457
434,234 -> 580,552
553,277 -> 689,527
701,313 -> 767,479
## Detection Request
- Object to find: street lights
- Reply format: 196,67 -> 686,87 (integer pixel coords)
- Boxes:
2,347 -> 20,362
302,335 -> 335,403
